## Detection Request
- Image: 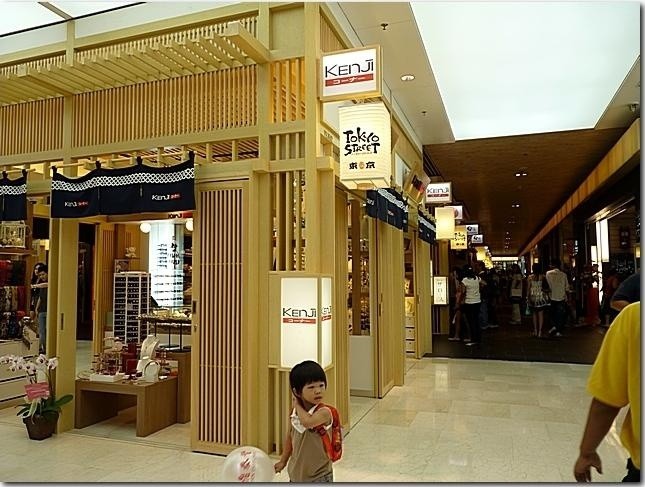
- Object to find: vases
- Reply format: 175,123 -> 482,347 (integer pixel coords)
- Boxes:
23,416 -> 57,441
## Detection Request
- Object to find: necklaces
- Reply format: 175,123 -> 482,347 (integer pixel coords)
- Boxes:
144,336 -> 156,351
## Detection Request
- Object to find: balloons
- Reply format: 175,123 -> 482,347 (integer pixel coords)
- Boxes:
215,445 -> 278,481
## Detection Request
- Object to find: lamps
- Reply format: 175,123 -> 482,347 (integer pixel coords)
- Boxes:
140,223 -> 151,234
185,218 -> 193,231
450,224 -> 468,250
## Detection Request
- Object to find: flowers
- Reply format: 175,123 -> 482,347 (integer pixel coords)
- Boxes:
0,353 -> 74,435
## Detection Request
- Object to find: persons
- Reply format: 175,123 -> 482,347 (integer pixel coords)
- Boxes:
274,360 -> 334,483
603,268 -> 620,329
30,264 -> 48,355
571,298 -> 640,484
611,268 -> 640,313
28,263 -> 44,326
448,258 -> 585,345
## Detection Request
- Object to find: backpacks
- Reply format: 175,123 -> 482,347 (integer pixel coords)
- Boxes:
313,403 -> 343,461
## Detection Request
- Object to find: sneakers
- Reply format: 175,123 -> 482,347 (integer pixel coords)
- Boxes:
509,320 -> 521,325
531,326 -> 563,339
482,324 -> 500,330
447,337 -> 477,346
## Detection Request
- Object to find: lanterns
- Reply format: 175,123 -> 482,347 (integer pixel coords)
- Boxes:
476,245 -> 495,269
450,223 -> 469,251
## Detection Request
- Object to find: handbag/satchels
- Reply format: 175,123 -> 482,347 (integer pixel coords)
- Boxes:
541,277 -> 550,292
525,301 -> 531,315
456,289 -> 465,304
511,288 -> 522,297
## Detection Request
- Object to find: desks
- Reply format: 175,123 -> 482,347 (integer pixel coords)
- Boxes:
117,350 -> 191,424
133,314 -> 191,351
75,375 -> 178,438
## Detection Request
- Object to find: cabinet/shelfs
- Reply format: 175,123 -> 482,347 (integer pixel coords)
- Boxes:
113,273 -> 152,344
405,316 -> 415,354
